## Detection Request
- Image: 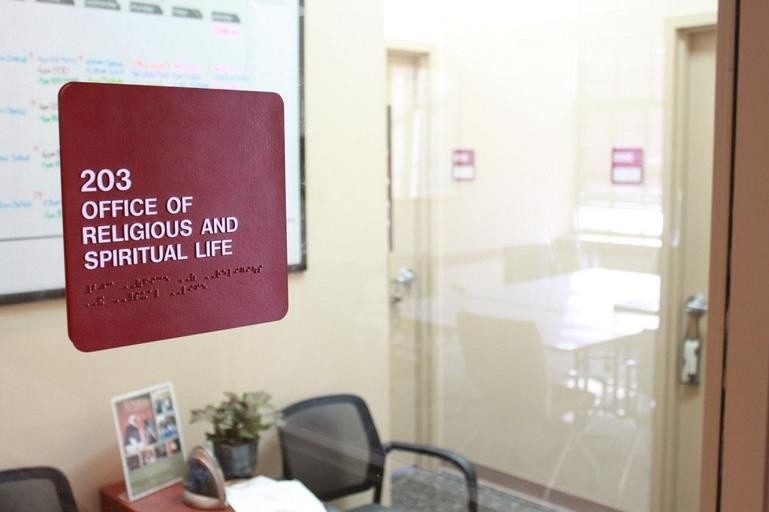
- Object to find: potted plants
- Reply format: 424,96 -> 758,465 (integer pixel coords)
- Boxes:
188,388 -> 286,481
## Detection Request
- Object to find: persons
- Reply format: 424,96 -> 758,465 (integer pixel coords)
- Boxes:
125,415 -> 142,469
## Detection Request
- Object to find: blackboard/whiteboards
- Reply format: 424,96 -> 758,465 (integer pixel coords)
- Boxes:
0,0 -> 310,306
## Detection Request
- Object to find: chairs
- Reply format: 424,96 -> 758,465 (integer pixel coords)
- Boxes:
274,394 -> 477,511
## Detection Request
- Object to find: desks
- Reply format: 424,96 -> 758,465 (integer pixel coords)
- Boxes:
99,475 -> 256,512
